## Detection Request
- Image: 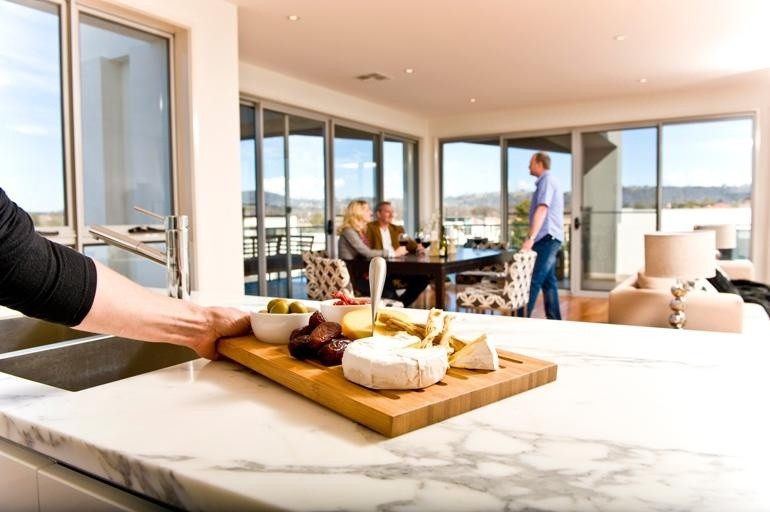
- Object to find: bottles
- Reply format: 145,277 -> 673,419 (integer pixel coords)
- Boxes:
438,225 -> 447,258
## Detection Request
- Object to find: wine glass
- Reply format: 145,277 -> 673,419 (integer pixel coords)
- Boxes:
474,235 -> 488,253
398,231 -> 432,258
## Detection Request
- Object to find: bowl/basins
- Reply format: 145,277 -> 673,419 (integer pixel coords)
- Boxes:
249,297 -> 383,344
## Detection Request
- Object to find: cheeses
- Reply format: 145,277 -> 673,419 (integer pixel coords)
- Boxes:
342,308 -> 410,343
447,333 -> 499,370
342,335 -> 449,390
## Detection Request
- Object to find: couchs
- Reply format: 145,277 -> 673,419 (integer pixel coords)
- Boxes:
607,258 -> 770,335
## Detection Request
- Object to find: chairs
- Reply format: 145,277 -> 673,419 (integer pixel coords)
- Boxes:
456,238 -> 510,284
301,248 -> 328,277
455,251 -> 538,317
307,257 -> 354,301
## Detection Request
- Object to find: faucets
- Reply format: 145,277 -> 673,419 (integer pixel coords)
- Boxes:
89,206 -> 190,300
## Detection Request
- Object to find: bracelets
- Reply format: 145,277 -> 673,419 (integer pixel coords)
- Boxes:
525,235 -> 536,241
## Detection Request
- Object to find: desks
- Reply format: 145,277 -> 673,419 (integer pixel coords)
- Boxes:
364,245 -> 516,311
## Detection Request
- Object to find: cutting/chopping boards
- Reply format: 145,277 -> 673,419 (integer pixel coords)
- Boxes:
215,333 -> 558,439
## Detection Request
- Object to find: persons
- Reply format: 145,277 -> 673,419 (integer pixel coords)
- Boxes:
366,202 -> 428,307
337,199 -> 409,298
517,152 -> 565,319
0,186 -> 253,361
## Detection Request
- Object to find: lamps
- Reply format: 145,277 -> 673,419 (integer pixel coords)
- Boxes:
696,225 -> 738,260
643,232 -> 716,328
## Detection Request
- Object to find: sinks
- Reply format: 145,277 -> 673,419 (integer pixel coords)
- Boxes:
0,336 -> 202,394
0,316 -> 100,354
